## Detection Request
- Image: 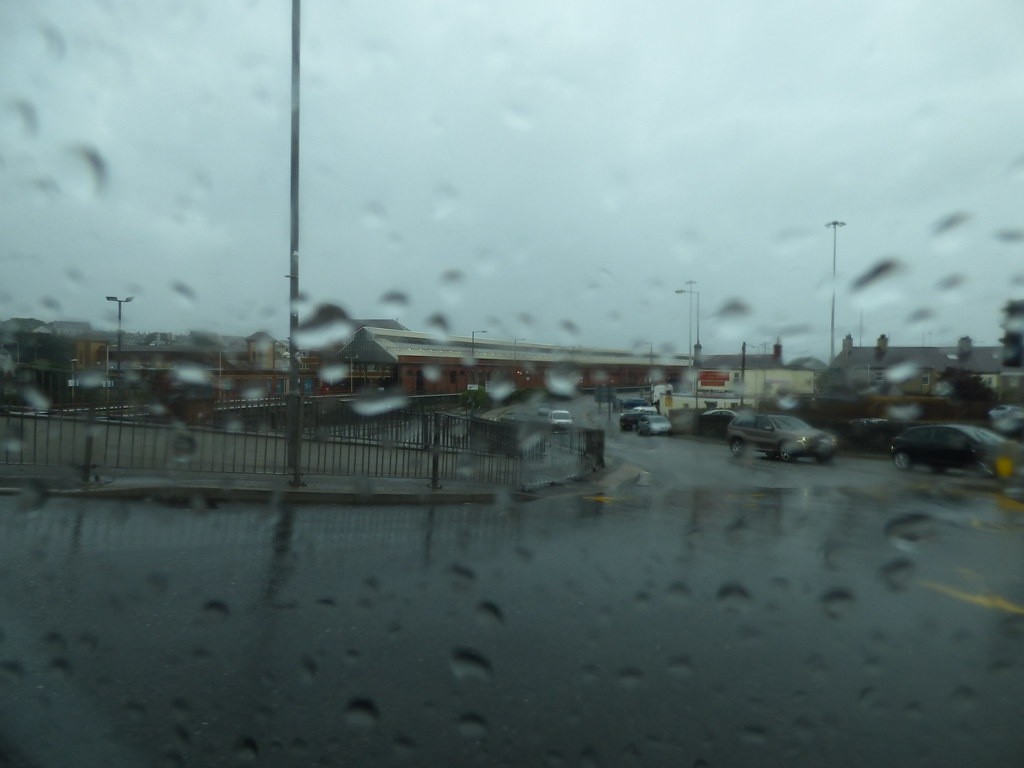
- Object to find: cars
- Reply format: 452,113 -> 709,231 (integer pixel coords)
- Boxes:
547,409 -> 575,433
621,406 -> 657,432
887,421 -> 1020,482
638,415 -> 675,436
703,409 -> 740,418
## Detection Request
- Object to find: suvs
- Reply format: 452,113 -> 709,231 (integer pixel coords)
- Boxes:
727,412 -> 839,467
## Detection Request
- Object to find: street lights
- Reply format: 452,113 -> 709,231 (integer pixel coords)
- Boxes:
105,344 -> 117,407
70,359 -> 78,402
822,220 -> 846,365
344,355 -> 358,392
105,296 -> 135,408
514,337 -> 527,373
471,329 -> 486,385
684,280 -> 698,378
674,289 -> 700,347
